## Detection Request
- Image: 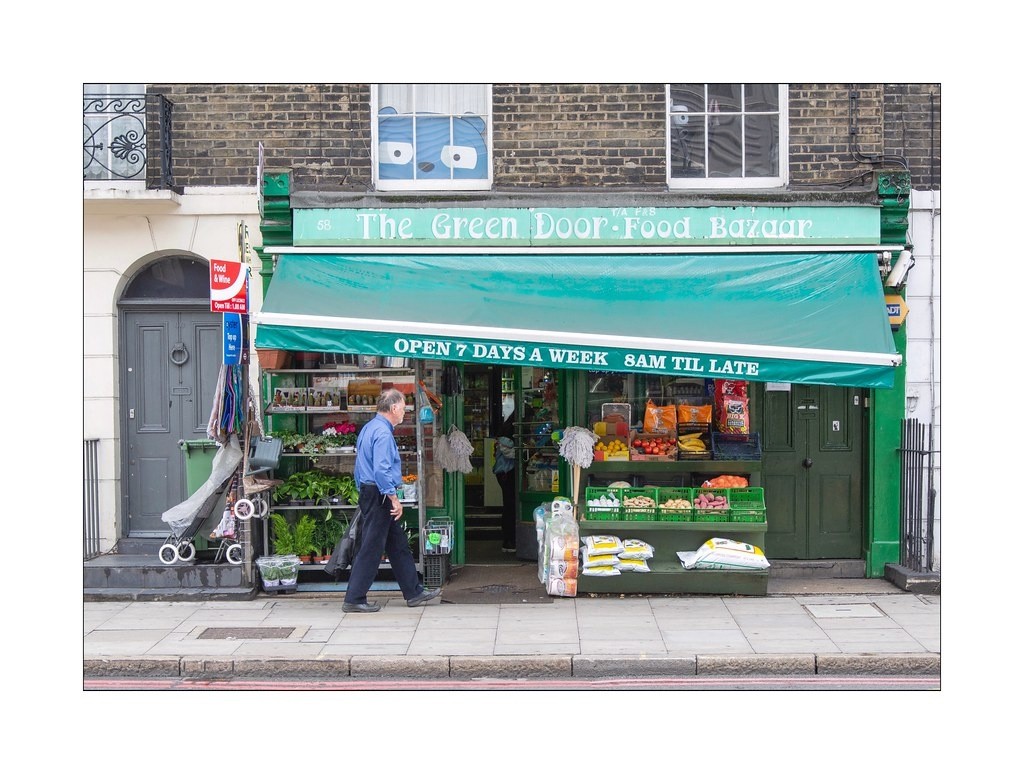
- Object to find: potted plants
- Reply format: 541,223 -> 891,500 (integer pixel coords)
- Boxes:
312,517 -> 323,561
255,554 -> 301,595
293,514 -> 311,562
323,519 -> 335,560
395,437 -> 416,451
273,469 -> 359,506
380,520 -> 420,564
266,428 -> 323,453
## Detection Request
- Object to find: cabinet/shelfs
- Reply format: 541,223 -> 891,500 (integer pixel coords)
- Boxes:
578,460 -> 768,596
463,362 -> 489,485
265,360 -> 425,575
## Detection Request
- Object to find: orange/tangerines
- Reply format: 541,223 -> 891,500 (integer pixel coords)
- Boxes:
595,440 -> 628,456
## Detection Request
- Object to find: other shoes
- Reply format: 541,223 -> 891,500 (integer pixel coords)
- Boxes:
501,544 -> 516,552
407,586 -> 441,607
341,601 -> 381,612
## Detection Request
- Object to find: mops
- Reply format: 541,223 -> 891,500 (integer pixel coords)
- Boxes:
557,426 -> 601,536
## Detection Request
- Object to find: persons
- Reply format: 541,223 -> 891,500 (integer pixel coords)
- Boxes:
492,405 -> 533,553
342,390 -> 442,613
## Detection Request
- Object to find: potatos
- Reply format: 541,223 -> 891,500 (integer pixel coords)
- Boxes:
622,492 -> 728,515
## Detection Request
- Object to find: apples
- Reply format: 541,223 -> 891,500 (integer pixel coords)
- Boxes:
633,435 -> 676,455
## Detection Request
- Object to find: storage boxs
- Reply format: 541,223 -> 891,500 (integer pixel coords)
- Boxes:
622,487 -> 657,521
585,487 -> 622,521
676,421 -> 712,460
346,379 -> 382,411
657,487 -> 694,522
393,383 -> 414,411
630,429 -> 678,460
693,487 -> 730,522
593,422 -> 630,461
306,387 -> 340,411
712,431 -> 762,461
422,551 -> 452,587
272,388 -> 306,411
729,487 -> 766,523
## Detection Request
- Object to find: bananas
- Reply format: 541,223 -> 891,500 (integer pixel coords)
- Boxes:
677,432 -> 706,451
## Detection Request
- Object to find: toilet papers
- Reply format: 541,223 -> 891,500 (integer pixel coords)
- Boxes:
534,509 -> 580,598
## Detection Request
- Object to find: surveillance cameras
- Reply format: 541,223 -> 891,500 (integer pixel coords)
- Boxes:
884,250 -> 912,288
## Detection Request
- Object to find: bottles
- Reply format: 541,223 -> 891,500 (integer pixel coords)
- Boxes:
464,376 -> 490,456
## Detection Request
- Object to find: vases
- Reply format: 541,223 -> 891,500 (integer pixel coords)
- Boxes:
402,482 -> 416,499
325,446 -> 354,453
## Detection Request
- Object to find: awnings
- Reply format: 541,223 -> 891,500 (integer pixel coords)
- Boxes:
253,251 -> 902,389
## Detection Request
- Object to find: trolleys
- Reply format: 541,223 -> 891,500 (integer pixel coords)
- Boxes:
159,451 -> 268,566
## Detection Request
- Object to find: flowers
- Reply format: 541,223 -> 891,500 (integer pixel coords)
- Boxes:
323,420 -> 355,447
402,474 -> 417,485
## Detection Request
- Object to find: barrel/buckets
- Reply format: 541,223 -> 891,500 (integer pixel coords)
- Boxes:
358,354 -> 381,368
383,357 -> 409,368
295,353 -> 321,368
